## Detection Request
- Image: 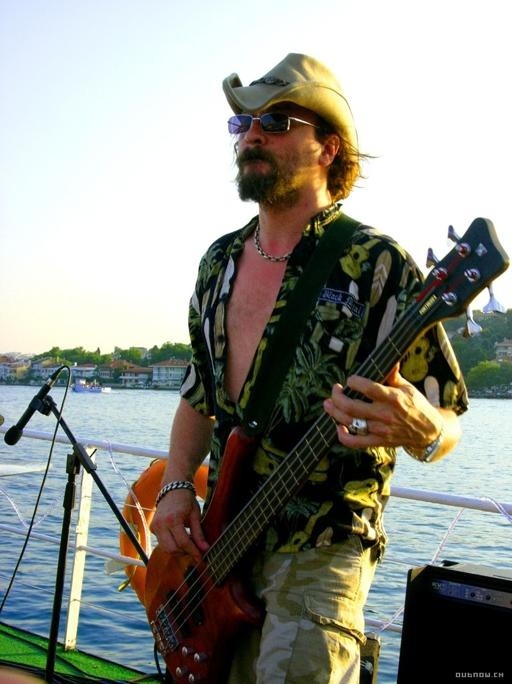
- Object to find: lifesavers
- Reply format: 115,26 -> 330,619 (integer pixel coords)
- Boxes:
120,461 -> 208,608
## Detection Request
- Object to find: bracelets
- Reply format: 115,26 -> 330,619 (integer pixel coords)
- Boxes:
155,479 -> 197,503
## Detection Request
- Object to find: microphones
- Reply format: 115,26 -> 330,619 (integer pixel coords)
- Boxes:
5,383 -> 52,446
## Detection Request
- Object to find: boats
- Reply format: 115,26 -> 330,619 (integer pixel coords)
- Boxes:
68,377 -> 113,394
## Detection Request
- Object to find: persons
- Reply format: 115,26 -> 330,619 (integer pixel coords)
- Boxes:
150,53 -> 470,683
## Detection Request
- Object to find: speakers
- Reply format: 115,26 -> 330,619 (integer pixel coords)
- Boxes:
396,556 -> 512,683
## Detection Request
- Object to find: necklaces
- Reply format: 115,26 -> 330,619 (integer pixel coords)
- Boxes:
252,221 -> 293,262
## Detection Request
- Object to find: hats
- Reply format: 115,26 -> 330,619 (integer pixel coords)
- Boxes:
222,53 -> 360,165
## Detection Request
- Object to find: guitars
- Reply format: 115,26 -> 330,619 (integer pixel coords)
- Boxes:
145,218 -> 510,684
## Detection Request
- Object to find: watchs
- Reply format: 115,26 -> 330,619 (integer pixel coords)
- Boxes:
402,428 -> 443,463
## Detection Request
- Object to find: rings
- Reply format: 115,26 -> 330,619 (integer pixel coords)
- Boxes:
349,417 -> 369,436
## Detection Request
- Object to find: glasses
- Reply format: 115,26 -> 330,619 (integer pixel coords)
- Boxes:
228,112 -> 320,134
